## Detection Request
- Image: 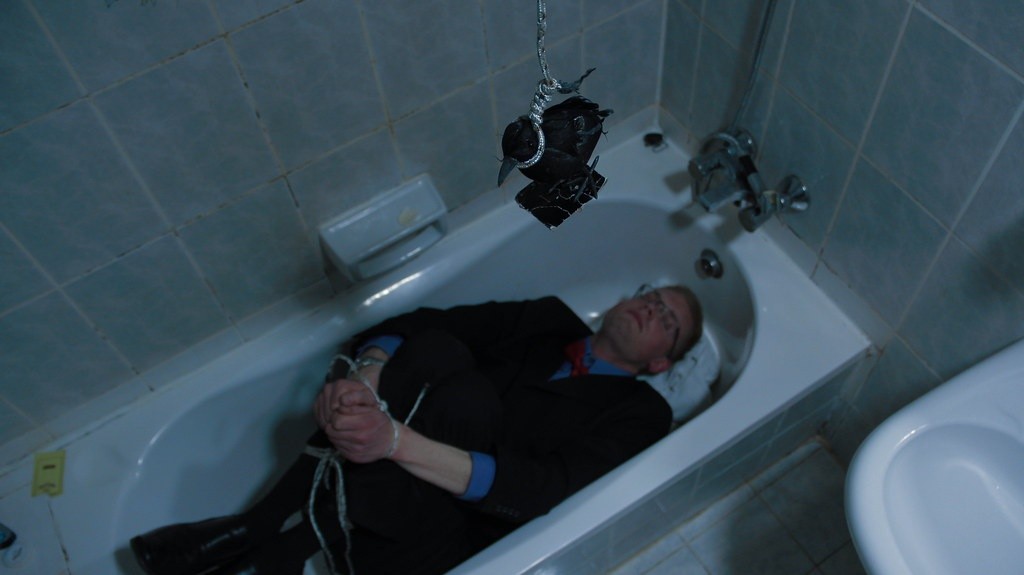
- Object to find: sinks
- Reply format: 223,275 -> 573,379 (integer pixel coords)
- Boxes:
843,330 -> 1023,575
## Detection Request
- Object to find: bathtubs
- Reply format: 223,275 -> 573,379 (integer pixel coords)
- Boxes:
0,120 -> 880,575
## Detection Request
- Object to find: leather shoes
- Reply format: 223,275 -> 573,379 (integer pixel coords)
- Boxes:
129,513 -> 304,575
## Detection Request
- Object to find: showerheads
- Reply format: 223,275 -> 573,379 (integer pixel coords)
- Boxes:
732,148 -> 775,232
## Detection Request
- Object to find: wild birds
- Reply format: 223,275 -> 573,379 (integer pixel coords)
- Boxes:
495,96 -> 614,190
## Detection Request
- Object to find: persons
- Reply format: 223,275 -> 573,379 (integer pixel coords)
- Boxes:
129,281 -> 704,573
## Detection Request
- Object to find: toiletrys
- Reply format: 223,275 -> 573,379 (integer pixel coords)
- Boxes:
0,520 -> 28,570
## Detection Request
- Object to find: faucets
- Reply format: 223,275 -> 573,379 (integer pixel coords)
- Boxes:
695,180 -> 750,216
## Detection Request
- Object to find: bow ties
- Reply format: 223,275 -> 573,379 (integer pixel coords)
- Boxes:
566,338 -> 589,377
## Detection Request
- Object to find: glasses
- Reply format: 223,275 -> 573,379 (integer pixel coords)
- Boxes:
632,283 -> 680,359
